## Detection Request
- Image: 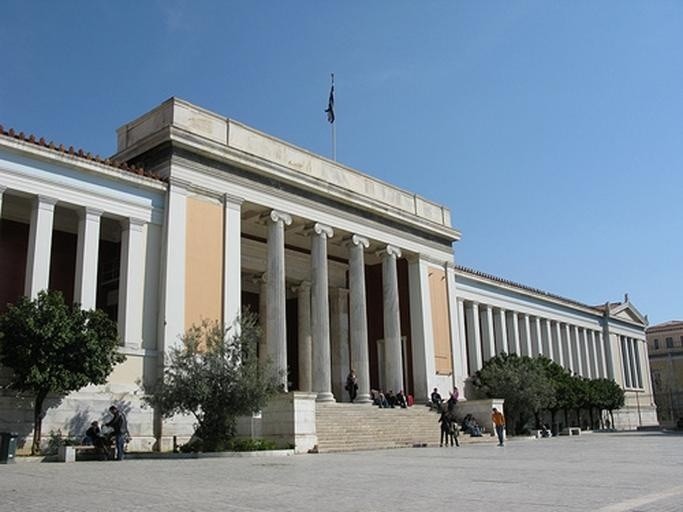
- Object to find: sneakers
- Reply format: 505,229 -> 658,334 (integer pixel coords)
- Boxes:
440,444 -> 460,447
497,443 -> 504,447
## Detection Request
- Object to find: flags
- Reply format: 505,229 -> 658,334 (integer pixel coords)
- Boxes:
325,72 -> 336,124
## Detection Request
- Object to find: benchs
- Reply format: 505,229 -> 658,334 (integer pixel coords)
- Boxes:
563,427 -> 582,435
58,444 -> 117,462
530,429 -> 552,438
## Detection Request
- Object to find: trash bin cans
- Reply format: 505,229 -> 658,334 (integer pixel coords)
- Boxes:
0,431 -> 19,464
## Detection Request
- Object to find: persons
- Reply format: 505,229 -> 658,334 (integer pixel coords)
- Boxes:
462,413 -> 483,435
370,389 -> 414,408
85,406 -> 128,461
345,367 -> 360,403
438,404 -> 460,447
432,388 -> 442,410
447,387 -> 459,410
491,407 -> 506,447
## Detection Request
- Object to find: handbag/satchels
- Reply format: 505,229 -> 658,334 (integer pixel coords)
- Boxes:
355,383 -> 358,389
121,432 -> 131,443
453,432 -> 458,437
345,384 -> 350,390
